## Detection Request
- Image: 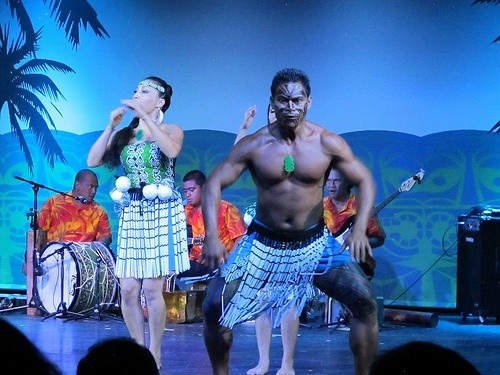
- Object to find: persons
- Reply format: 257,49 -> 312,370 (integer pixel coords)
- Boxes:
85,76 -> 191,374
200,69 -> 387,375
180,169 -> 247,287
75,337 -> 160,375
0,318 -> 64,375
368,339 -> 480,375
22,168 -> 112,278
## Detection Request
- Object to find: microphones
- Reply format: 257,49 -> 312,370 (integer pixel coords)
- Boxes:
78,196 -> 87,203
33,264 -> 43,276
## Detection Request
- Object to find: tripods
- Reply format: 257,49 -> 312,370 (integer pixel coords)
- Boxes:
0,176 -> 125,323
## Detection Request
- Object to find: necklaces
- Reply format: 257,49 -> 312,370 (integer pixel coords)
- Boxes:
280,129 -> 300,172
136,129 -> 144,141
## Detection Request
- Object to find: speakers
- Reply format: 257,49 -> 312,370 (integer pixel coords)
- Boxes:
456,215 -> 500,317
161,289 -> 207,324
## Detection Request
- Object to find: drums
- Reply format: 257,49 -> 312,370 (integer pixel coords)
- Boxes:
31,239 -> 117,319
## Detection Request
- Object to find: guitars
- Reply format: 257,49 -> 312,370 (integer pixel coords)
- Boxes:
333,168 -> 425,249
